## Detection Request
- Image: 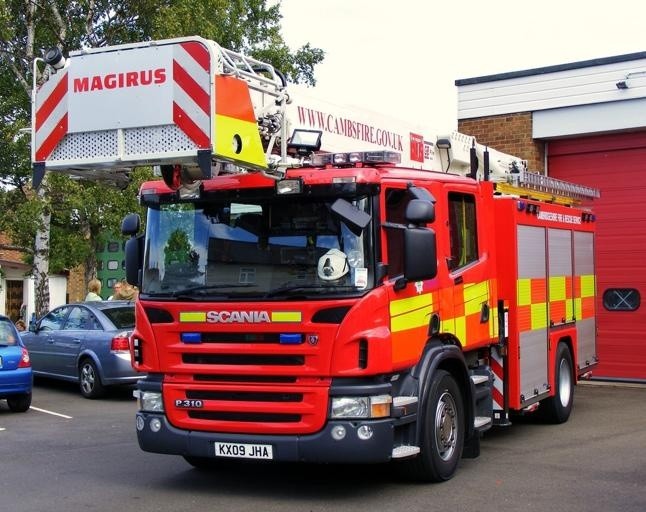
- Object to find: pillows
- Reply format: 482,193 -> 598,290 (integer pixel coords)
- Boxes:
317,248 -> 351,281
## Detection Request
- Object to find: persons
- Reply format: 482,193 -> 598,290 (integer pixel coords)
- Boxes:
111,278 -> 138,302
108,282 -> 123,301
84,278 -> 102,301
15,320 -> 25,331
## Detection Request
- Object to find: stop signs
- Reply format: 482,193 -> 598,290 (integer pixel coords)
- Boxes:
30,35 -> 600,482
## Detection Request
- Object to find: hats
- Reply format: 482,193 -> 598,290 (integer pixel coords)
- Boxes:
0,299 -> 148,412
